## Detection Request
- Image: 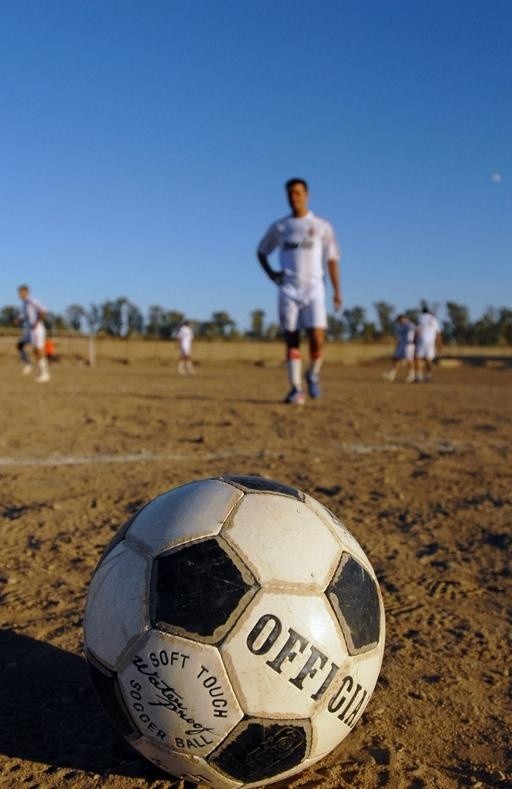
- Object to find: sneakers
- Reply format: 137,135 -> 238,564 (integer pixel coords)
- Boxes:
20,362 -> 51,383
381,369 -> 432,384
177,365 -> 197,375
284,386 -> 306,405
305,371 -> 321,399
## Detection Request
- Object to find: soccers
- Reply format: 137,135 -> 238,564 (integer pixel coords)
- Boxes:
83,475 -> 386,789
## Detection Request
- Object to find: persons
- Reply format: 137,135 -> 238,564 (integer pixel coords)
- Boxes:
257,178 -> 341,403
382,315 -> 418,384
12,286 -> 52,383
176,320 -> 195,375
413,305 -> 444,383
